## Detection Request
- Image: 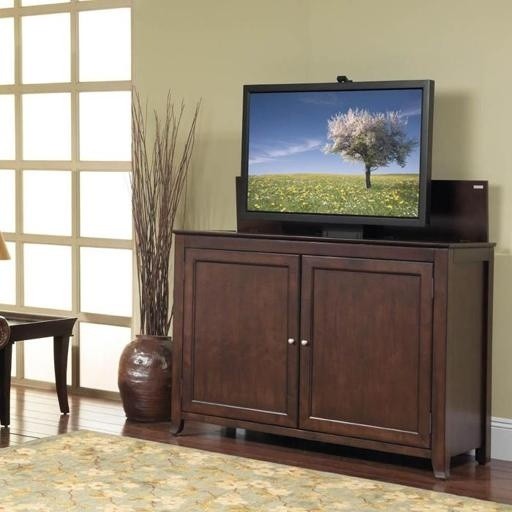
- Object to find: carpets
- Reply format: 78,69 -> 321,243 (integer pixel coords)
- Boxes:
0,426 -> 511,512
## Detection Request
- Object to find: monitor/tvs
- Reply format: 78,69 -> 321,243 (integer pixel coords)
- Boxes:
238,79 -> 436,240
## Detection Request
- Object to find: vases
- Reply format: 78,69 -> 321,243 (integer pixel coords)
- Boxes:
114,333 -> 176,425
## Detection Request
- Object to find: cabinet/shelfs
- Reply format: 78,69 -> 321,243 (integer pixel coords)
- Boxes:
167,227 -> 495,479
